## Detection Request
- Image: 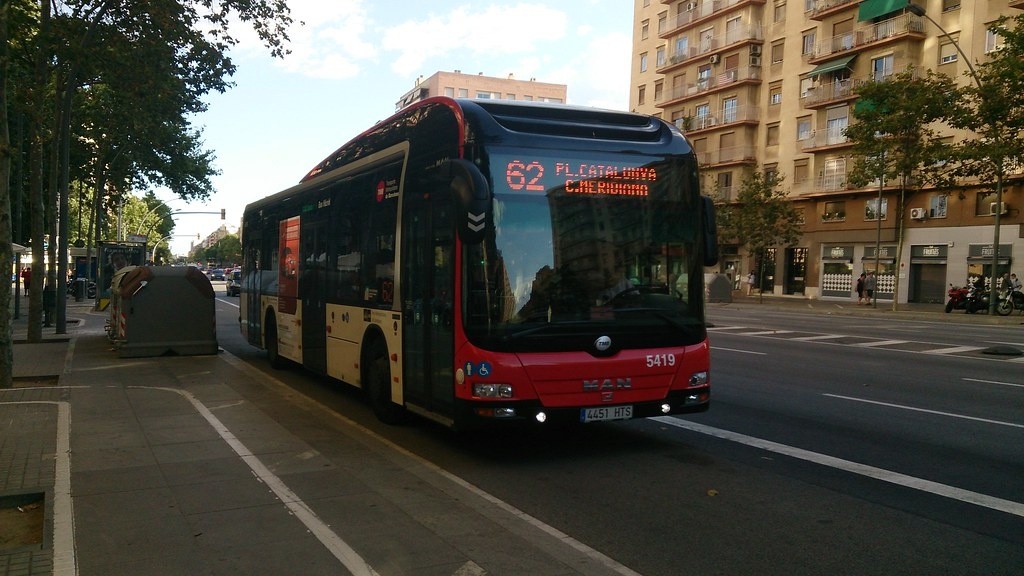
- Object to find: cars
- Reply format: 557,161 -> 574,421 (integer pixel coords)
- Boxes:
225,270 -> 241,297
211,270 -> 224,281
12,275 -> 16,283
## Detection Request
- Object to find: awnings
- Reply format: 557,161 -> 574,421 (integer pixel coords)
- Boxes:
861,256 -> 896,264
822,255 -> 853,264
806,54 -> 857,79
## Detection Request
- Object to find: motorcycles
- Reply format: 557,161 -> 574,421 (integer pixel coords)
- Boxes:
65,278 -> 96,299
965,283 -> 1000,315
945,282 -> 969,313
994,284 -> 1024,316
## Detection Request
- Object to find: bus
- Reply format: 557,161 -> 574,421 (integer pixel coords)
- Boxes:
239,97 -> 721,433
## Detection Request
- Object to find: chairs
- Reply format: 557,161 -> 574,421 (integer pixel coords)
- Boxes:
287,261 -> 360,305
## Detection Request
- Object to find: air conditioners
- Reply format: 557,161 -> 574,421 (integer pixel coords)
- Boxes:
989,202 -> 1008,214
750,44 -> 761,54
910,208 -> 925,219
708,118 -> 716,126
687,2 -> 696,12
710,54 -> 720,64
750,56 -> 761,65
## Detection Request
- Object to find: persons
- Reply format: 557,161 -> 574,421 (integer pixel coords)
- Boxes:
855,272 -> 875,305
964,276 -> 985,314
581,228 -> 639,313
104,251 -> 129,292
1001,273 -> 1022,292
746,270 -> 755,295
25,268 -> 31,297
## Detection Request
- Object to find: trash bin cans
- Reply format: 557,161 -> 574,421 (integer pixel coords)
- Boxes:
75,276 -> 88,302
676,273 -> 732,303
105,264 -> 220,359
